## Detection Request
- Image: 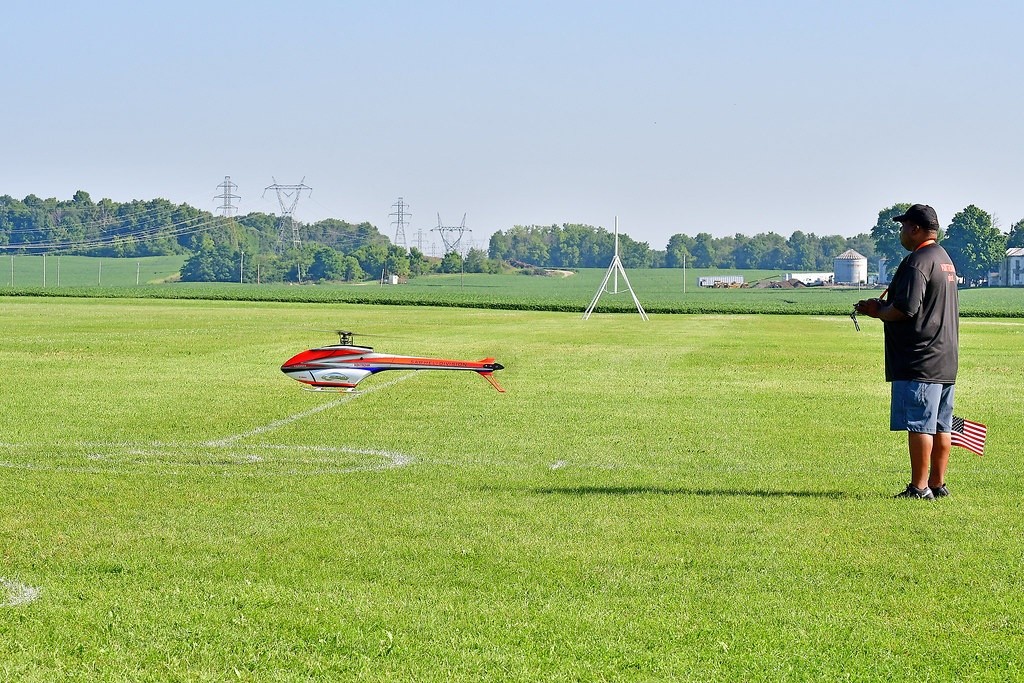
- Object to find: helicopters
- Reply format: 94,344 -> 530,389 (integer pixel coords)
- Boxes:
281,327 -> 505,393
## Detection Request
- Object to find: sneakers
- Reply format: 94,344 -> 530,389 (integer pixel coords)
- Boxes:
892,484 -> 951,501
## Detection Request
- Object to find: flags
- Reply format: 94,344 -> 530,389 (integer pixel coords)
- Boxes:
951,415 -> 987,457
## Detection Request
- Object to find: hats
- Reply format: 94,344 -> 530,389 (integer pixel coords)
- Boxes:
893,204 -> 939,231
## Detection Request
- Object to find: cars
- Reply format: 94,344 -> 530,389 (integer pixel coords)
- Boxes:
978,282 -> 988,287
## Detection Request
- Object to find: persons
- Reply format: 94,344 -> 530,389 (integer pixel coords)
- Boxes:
854,204 -> 959,501
